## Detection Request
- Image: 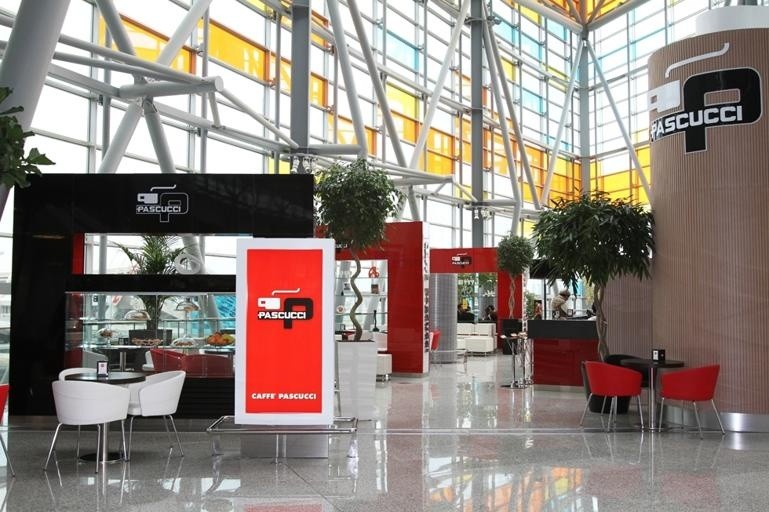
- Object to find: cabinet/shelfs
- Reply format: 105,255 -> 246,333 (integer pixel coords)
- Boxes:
335,260 -> 387,333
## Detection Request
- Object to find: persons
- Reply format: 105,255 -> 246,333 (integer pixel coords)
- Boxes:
458,304 -> 465,320
535,301 -> 542,320
552,290 -> 572,318
486,305 -> 494,321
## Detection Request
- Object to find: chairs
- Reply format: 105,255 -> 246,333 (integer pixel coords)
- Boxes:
579,354 -> 726,440
83,342 -> 235,378
42,365 -> 188,474
457,322 -> 499,358
0,382 -> 17,477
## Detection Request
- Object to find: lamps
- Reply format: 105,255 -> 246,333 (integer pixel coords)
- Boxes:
174,295 -> 201,314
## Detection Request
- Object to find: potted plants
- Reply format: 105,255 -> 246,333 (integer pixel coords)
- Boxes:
310,154 -> 410,422
527,183 -> 656,413
106,233 -> 200,371
493,229 -> 535,355
